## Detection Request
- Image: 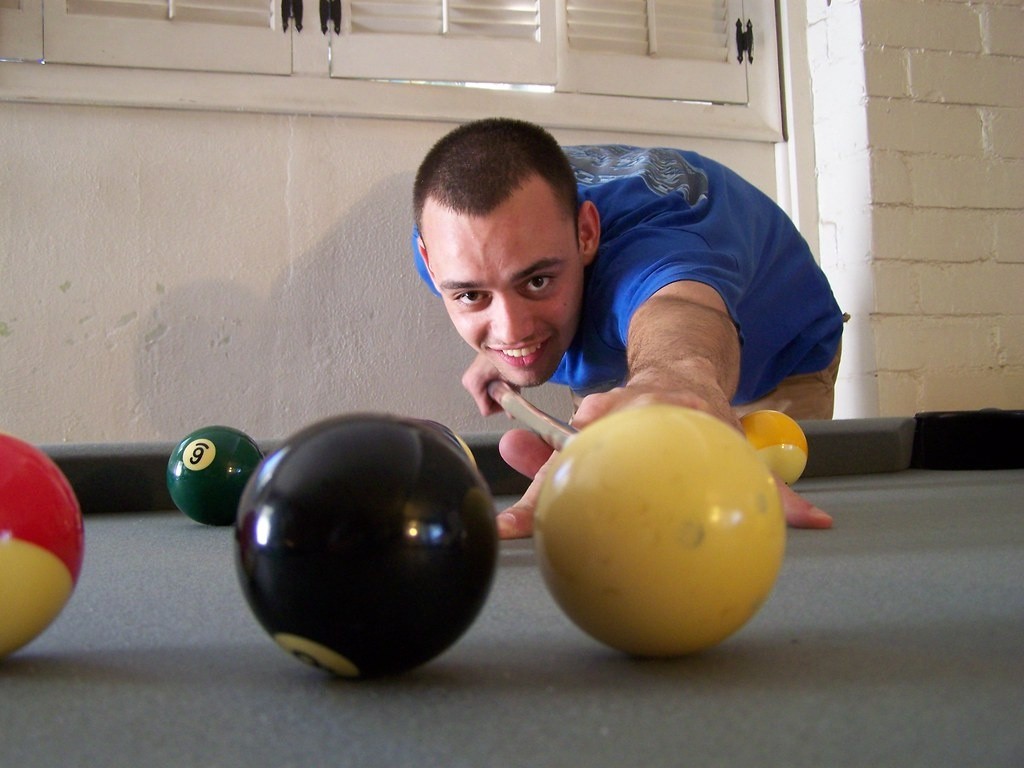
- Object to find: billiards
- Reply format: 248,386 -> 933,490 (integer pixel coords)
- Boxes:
225,412 -> 502,680
0,434 -> 88,657
736,407 -> 808,488
166,428 -> 261,528
535,402 -> 788,659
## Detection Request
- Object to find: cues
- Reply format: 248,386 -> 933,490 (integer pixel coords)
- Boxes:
484,379 -> 579,445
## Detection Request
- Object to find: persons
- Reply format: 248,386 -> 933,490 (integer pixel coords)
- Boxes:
410,118 -> 848,540
